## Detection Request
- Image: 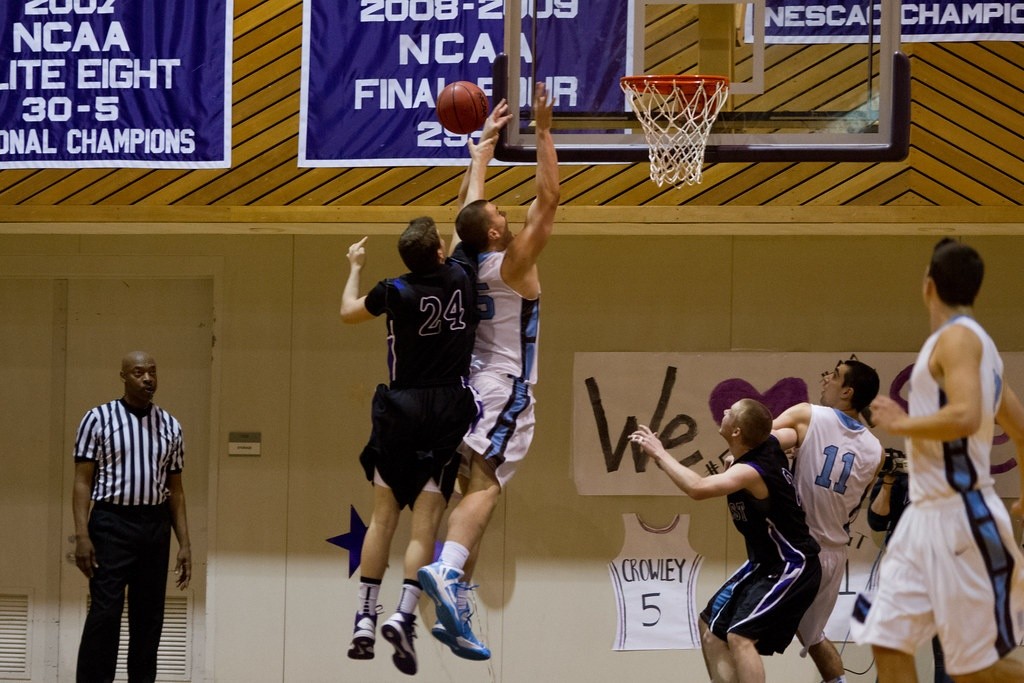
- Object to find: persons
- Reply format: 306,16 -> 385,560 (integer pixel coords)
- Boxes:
71,351 -> 192,683
341,82 -> 558,675
850,237 -> 1024,683
626,359 -> 890,682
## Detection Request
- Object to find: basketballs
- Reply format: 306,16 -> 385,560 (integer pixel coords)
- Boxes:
435,80 -> 490,136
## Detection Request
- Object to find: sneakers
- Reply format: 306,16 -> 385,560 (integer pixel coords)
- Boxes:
418,561 -> 464,638
347,605 -> 377,661
381,612 -> 420,675
428,611 -> 493,661
